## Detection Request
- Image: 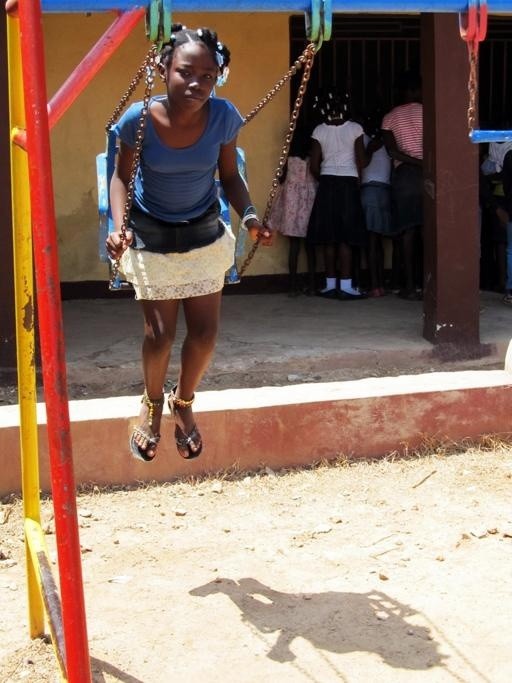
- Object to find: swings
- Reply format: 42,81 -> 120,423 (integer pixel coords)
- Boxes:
95,44 -> 314,293
467,39 -> 512,144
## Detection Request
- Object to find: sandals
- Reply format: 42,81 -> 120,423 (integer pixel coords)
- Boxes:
166,384 -> 202,461
130,387 -> 165,463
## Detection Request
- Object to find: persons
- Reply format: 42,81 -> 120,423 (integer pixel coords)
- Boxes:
267,75 -> 422,300
106,23 -> 278,459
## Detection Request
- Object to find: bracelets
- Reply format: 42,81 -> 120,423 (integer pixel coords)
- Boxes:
240,206 -> 260,231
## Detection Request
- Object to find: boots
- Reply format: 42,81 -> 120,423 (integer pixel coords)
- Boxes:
319,277 -> 336,293
339,279 -> 361,296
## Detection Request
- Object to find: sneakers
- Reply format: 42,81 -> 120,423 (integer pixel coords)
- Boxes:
288,286 -> 315,297
362,284 -> 423,300
